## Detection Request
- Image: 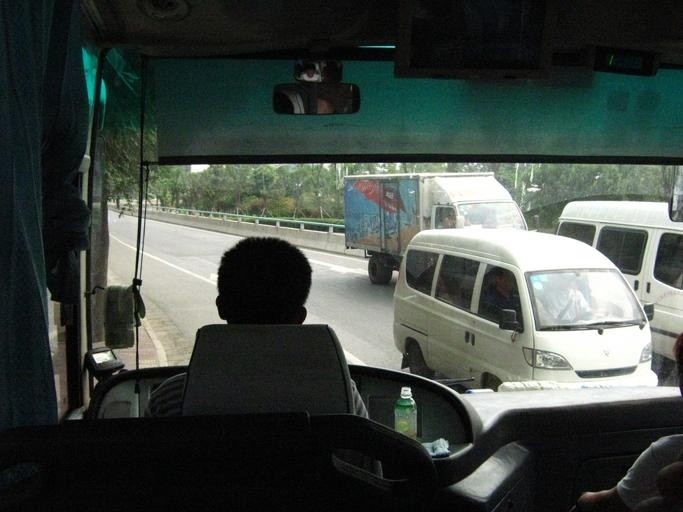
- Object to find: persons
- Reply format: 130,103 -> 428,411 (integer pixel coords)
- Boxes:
437,215 -> 453,229
143,238 -> 369,418
316,91 -> 337,113
547,276 -> 599,322
299,62 -> 322,83
479,268 -> 522,327
568,329 -> 683,511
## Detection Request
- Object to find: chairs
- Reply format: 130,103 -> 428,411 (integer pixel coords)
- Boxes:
183,324 -> 354,413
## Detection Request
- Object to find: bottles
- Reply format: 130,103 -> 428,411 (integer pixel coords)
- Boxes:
393,385 -> 419,441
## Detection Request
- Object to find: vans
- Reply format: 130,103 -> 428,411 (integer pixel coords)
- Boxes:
556,201 -> 682,363
392,229 -> 657,388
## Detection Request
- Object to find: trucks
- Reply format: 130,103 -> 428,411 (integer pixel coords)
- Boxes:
344,173 -> 529,285
